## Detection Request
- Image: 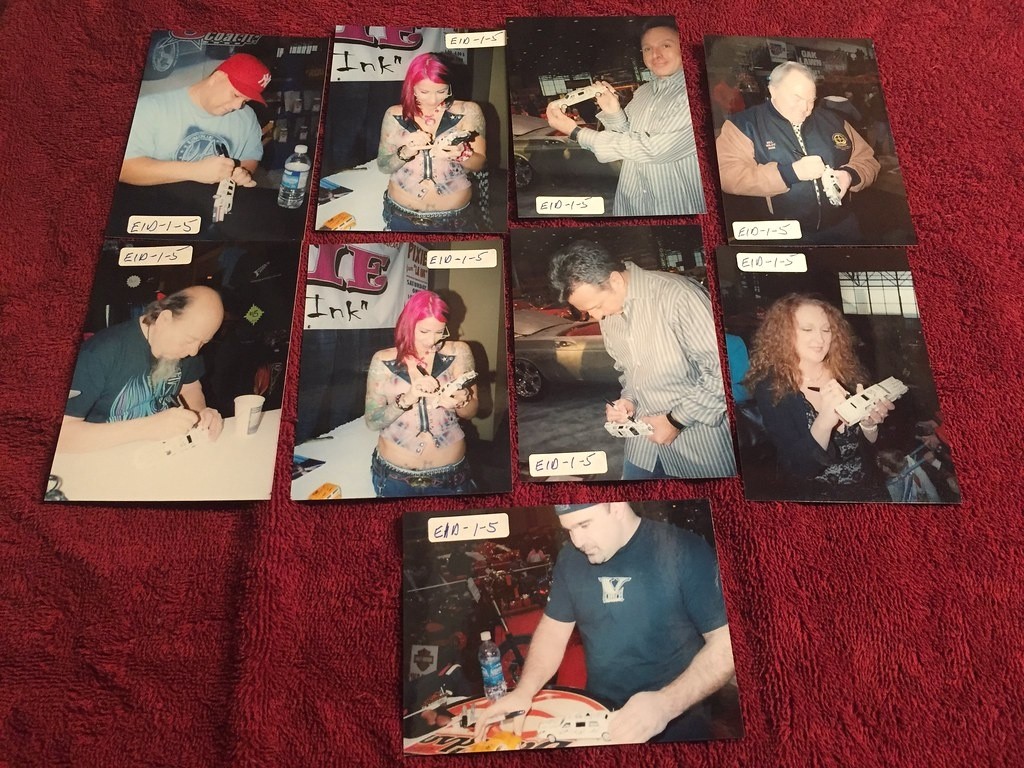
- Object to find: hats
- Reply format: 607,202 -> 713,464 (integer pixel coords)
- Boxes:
216,52 -> 272,106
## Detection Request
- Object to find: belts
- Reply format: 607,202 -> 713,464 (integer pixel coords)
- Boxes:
385,203 -> 472,227
378,460 -> 469,486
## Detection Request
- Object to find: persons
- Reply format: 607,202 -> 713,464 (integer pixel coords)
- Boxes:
712,59 -> 884,243
546,239 -> 738,482
58,284 -> 224,455
377,52 -> 487,232
545,16 -> 706,216
362,290 -> 478,498
118,53 -> 272,189
727,293 -> 961,505
399,500 -> 746,745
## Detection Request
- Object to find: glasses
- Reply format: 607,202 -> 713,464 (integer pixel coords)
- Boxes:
413,84 -> 452,99
415,325 -> 450,339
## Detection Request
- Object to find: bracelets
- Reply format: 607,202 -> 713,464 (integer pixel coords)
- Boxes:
667,412 -> 684,430
395,394 -> 413,411
568,126 -> 582,143
458,389 -> 473,407
396,145 -> 415,162
458,146 -> 474,161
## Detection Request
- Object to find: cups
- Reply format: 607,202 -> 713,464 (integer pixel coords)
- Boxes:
234,395 -> 266,435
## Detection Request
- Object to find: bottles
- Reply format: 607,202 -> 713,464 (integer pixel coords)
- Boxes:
277,145 -> 310,210
478,631 -> 507,702
